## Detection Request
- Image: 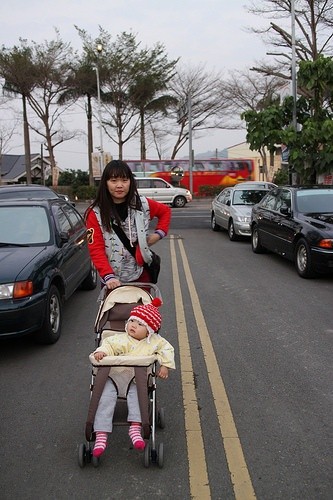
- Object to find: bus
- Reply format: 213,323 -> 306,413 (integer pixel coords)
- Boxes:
123,159 -> 256,196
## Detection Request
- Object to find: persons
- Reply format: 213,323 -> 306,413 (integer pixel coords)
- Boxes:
93,297 -> 176,457
84,160 -> 171,297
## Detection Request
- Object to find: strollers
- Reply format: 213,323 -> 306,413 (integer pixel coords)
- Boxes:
77,282 -> 165,469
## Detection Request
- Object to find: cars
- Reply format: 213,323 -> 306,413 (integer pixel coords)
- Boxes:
233,181 -> 279,192
210,186 -> 272,242
57,193 -> 76,207
249,183 -> 333,279
0,183 -> 98,344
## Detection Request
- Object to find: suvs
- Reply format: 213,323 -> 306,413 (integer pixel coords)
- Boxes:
133,177 -> 193,208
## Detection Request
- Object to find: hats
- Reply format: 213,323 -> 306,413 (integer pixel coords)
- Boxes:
125,298 -> 162,344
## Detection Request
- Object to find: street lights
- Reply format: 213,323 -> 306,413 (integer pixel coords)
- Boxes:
92,66 -> 104,174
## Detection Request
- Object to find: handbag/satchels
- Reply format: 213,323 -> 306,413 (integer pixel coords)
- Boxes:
149,249 -> 160,285
